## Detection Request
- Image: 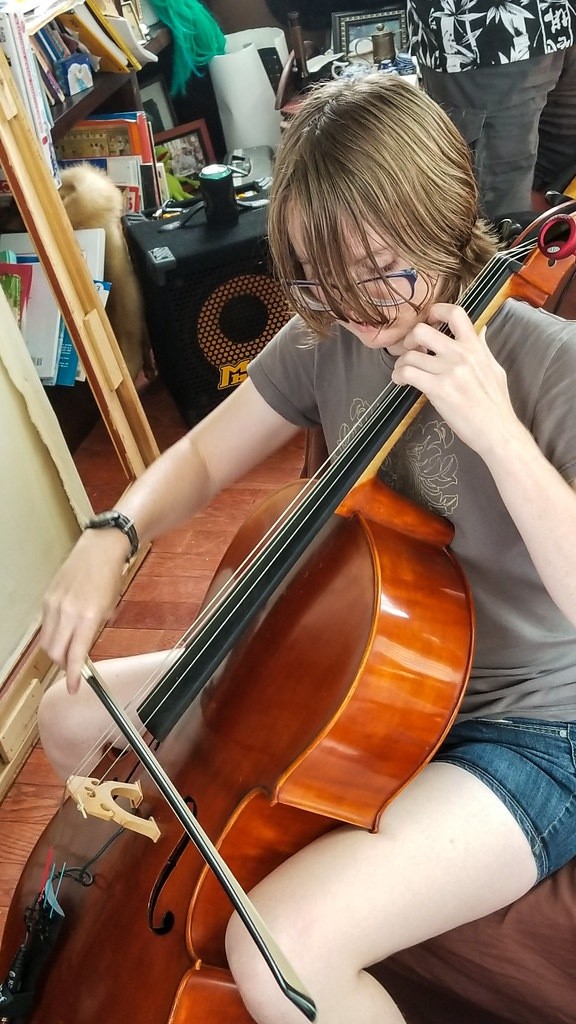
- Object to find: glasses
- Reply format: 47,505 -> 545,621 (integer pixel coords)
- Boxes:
282,268 -> 418,312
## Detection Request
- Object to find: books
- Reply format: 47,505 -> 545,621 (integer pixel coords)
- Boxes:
0,0 -> 170,386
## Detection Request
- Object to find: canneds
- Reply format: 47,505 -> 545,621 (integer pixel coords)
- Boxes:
198,163 -> 239,228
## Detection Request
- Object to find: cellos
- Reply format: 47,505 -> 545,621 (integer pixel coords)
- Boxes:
0,186 -> 576,1024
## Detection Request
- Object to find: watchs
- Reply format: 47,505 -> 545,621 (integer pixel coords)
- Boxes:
83,509 -> 139,562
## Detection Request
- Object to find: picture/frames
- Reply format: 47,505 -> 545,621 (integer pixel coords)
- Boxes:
153,116 -> 217,194
331,2 -> 413,69
139,72 -> 179,132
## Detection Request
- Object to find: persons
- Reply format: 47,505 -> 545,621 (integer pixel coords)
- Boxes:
38,75 -> 576,1024
172,141 -> 204,177
406,0 -> 576,219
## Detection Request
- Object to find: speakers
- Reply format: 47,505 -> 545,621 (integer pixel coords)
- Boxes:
125,188 -> 300,429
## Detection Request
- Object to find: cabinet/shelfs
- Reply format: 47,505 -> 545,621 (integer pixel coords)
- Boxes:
0,0 -> 220,454
